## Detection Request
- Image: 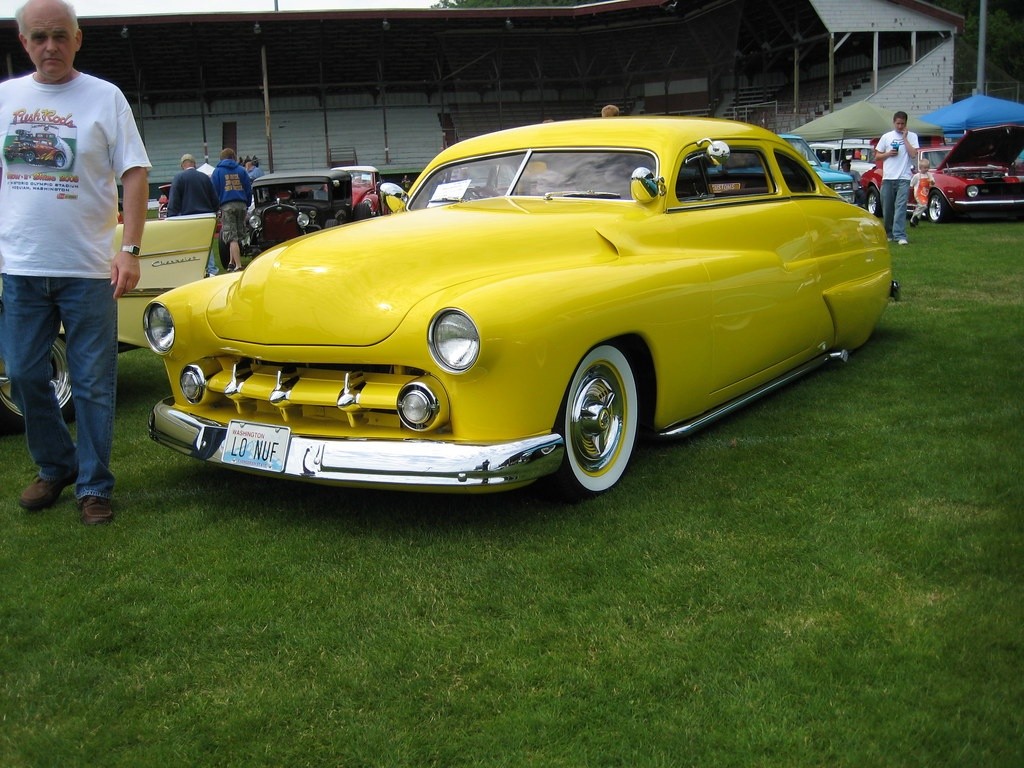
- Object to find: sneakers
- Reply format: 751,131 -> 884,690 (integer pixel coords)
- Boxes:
18,474 -> 79,509
78,495 -> 114,526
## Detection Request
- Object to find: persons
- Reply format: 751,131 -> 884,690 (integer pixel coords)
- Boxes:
910,159 -> 935,228
841,160 -> 866,207
875,111 -> 920,245
165,147 -> 264,277
601,104 -> 619,117
0,0 -> 152,526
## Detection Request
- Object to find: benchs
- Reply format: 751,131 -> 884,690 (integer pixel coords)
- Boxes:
448,62 -> 894,143
117,96 -> 445,185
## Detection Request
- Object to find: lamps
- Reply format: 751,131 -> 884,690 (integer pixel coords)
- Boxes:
119,27 -> 129,39
669,0 -> 678,12
253,20 -> 263,36
504,17 -> 514,29
382,18 -> 391,33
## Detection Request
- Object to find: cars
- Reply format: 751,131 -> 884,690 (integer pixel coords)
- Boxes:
796,137 -> 875,182
858,121 -> 1024,225
703,133 -> 856,206
141,116 -> 902,503
0,160 -> 393,433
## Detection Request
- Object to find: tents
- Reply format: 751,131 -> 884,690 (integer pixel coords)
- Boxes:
785,95 -> 1024,171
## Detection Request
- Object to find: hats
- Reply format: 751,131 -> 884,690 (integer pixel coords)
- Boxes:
842,160 -> 851,167
180,153 -> 195,165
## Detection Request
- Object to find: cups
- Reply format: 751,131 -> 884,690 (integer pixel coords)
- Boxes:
893,144 -> 899,151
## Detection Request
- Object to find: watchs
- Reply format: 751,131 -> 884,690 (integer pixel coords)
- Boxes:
119,245 -> 142,259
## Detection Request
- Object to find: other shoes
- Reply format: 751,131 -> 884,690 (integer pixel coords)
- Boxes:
898,239 -> 908,245
234,267 -> 244,272
887,237 -> 892,241
227,263 -> 234,271
